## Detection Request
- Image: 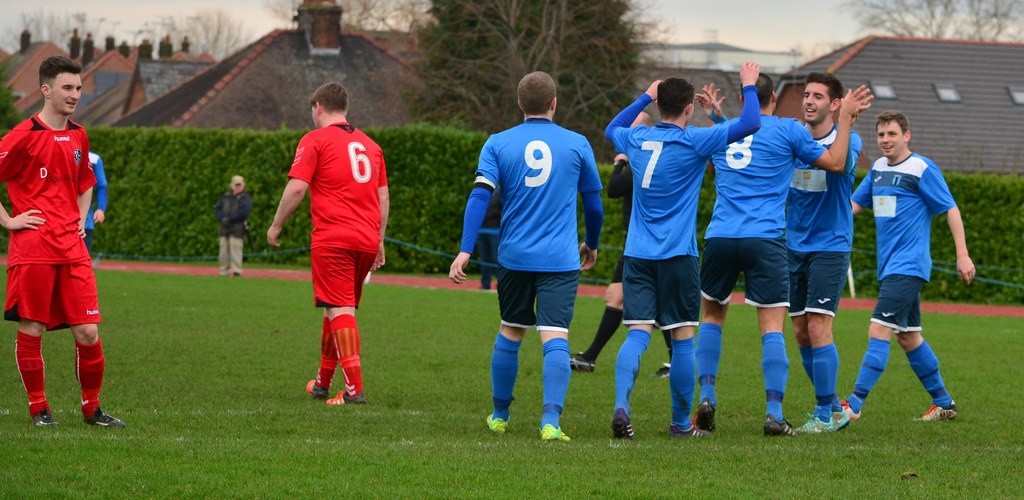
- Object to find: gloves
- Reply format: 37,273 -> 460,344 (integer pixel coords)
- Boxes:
221,217 -> 229,227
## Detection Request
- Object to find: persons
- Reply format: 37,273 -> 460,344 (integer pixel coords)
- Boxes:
0,55 -> 126,429
604,62 -> 761,437
83,152 -> 107,251
447,72 -> 604,441
267,81 -> 389,404
570,112 -> 672,377
215,176 -> 251,275
694,73 -> 861,433
838,111 -> 976,420
692,73 -> 874,435
477,194 -> 499,289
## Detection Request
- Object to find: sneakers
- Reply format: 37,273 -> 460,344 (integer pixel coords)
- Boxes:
83,407 -> 125,429
764,414 -> 798,438
326,390 -> 366,407
569,352 -> 595,372
307,379 -> 329,400
794,412 -> 836,434
693,400 -> 715,434
841,400 -> 861,420
487,414 -> 510,435
651,362 -> 672,379
830,409 -> 852,431
33,406 -> 60,427
669,420 -> 711,439
539,424 -> 570,442
610,408 -> 636,440
913,400 -> 958,422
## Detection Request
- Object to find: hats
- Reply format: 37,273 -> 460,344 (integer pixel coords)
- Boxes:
231,176 -> 244,186
